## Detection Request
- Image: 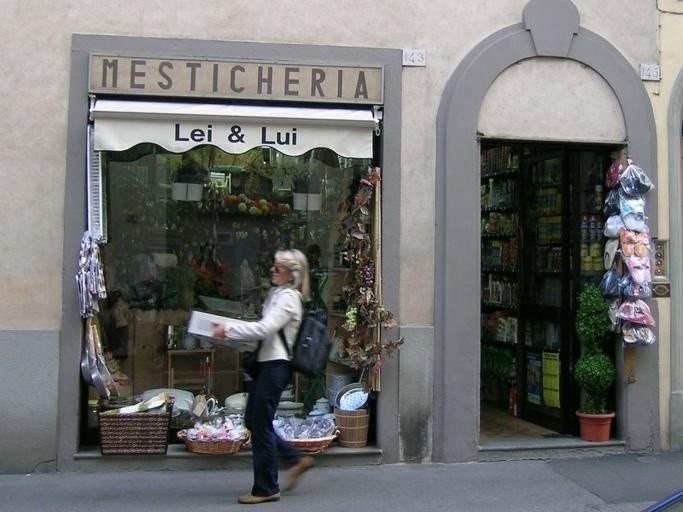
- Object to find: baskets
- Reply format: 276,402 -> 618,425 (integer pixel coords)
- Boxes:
95,396 -> 175,457
177,426 -> 250,455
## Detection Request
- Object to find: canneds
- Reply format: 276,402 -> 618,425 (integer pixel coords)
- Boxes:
579,214 -> 605,272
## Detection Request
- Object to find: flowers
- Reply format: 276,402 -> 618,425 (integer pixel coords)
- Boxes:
335,166 -> 404,387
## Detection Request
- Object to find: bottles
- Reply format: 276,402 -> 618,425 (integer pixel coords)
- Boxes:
481,143 -> 520,418
199,354 -> 210,375
165,325 -> 176,349
532,185 -> 562,307
308,395 -> 330,419
577,215 -> 603,242
328,328 -> 347,362
593,185 -> 604,202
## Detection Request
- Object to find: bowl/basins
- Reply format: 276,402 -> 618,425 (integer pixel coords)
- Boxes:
326,373 -> 357,406
223,384 -> 304,417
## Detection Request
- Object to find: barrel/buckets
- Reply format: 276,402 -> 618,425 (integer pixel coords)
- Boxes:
335,406 -> 369,448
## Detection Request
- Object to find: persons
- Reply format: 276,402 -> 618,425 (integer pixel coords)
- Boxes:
211,248 -> 315,506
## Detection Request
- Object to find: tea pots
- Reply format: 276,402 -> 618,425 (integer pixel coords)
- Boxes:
190,395 -> 215,416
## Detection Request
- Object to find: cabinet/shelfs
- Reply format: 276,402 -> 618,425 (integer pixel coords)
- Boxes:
168,347 -> 239,398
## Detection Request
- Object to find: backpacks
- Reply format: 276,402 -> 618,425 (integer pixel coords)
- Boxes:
277,287 -> 333,378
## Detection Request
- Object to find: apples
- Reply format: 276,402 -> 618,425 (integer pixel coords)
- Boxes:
238,199 -> 269,216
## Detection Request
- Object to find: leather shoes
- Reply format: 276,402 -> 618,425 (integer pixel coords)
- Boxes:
238,489 -> 281,504
281,455 -> 316,492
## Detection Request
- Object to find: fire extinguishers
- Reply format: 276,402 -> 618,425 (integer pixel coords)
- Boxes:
509,383 -> 517,416
